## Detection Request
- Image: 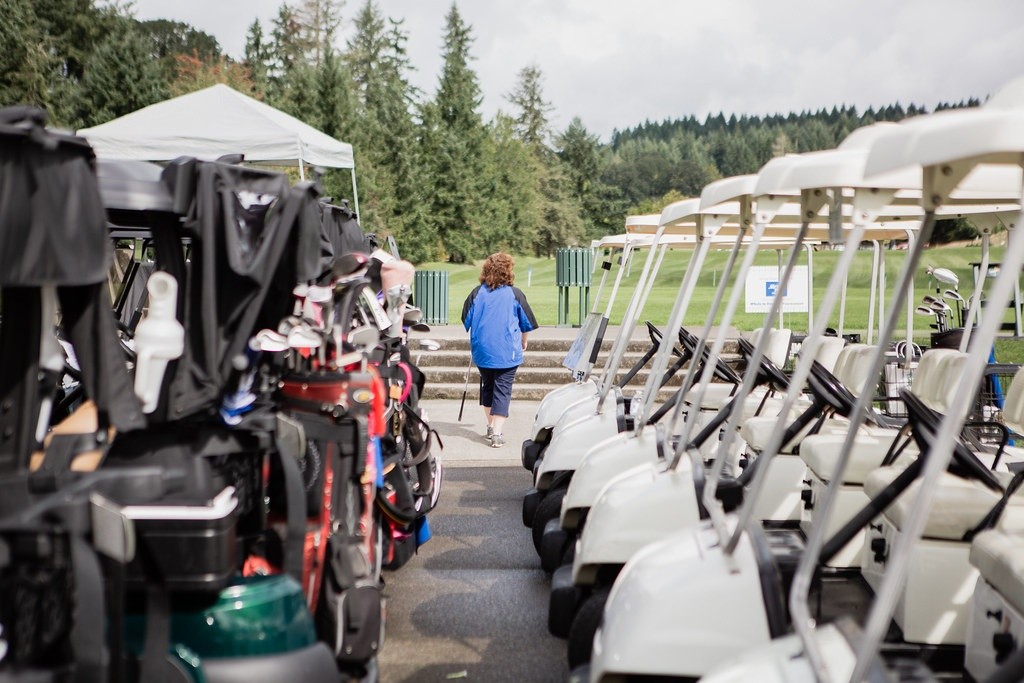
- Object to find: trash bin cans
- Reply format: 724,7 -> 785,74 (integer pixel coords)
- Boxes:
414,270 -> 447,325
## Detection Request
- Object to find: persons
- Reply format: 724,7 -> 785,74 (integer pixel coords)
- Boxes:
461,253 -> 539,447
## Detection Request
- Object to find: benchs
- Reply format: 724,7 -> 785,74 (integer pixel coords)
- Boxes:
685,326 -> 793,408
720,336 -> 847,425
799,349 -> 971,485
865,365 -> 1024,541
738,344 -> 875,453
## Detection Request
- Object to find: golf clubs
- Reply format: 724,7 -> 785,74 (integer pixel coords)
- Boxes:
915,268 -> 975,333
457,354 -> 474,423
243,285 -> 338,371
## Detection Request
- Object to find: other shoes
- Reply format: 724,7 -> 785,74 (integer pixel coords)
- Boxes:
486,427 -> 493,438
492,434 -> 505,447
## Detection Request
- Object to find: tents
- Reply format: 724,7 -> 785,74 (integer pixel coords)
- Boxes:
77,81 -> 360,226
